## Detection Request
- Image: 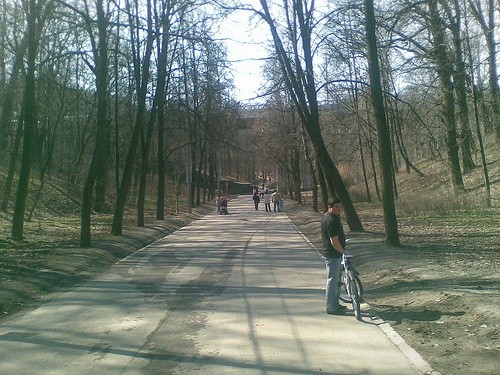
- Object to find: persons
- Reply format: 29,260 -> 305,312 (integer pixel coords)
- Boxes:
263,192 -> 271,212
253,188 -> 256,195
220,198 -> 227,215
270,190 -> 281,212
321,197 -> 349,315
253,193 -> 260,210
265,189 -> 268,194
216,195 -> 220,213
262,188 -> 264,196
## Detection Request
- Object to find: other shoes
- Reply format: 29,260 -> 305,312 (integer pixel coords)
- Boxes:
327,309 -> 347,315
339,305 -> 347,309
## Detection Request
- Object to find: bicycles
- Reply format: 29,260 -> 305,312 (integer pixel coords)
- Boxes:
338,237 -> 364,321
